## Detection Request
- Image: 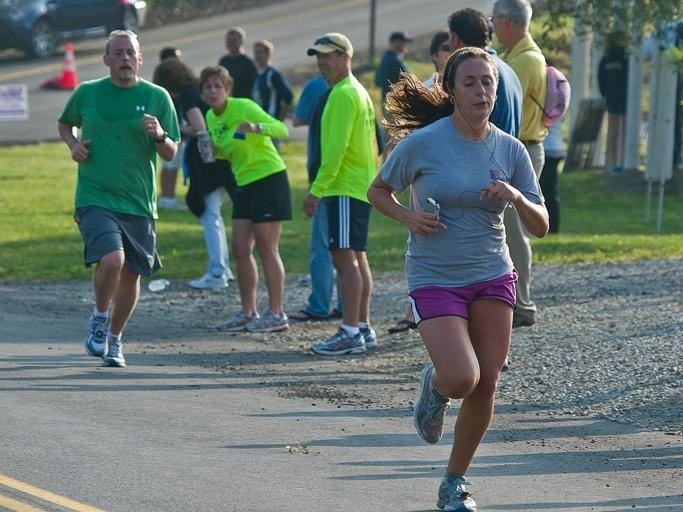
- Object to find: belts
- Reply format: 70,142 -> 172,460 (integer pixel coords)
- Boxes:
521,140 -> 539,147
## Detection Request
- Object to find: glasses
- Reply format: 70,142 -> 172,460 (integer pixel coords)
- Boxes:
314,38 -> 345,51
110,28 -> 137,39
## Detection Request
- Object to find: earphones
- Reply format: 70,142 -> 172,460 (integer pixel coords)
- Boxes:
450,85 -> 455,93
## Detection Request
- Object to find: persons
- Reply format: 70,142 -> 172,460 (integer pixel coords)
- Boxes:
368,46 -> 550,512
219,27 -> 293,150
58,30 -> 181,368
387,28 -> 452,332
151,48 -> 292,332
377,32 -> 412,113
289,33 -> 377,354
493,0 -> 571,325
448,8 -> 522,139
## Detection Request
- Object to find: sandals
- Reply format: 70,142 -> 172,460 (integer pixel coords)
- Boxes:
389,319 -> 416,334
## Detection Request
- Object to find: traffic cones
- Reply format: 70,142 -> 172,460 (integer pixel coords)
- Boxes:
54,42 -> 81,92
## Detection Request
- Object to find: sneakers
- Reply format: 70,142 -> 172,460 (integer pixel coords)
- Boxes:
415,364 -> 449,444
189,266 -> 234,289
436,475 -> 476,512
247,311 -> 289,332
217,312 -> 260,332
310,325 -> 378,356
86,311 -> 126,368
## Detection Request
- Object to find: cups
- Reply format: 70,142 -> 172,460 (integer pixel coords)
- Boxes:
195,131 -> 215,164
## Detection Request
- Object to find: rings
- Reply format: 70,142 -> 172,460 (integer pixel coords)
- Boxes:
148,124 -> 151,128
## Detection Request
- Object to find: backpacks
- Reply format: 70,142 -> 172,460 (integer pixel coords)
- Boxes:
512,47 -> 571,125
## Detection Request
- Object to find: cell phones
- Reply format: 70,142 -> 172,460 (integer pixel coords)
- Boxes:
424,197 -> 440,228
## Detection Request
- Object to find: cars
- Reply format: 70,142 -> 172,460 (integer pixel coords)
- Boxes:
0,1 -> 147,61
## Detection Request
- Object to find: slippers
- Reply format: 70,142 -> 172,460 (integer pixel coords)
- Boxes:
292,307 -> 338,321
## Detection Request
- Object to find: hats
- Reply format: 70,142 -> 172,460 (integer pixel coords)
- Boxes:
307,33 -> 353,59
390,34 -> 410,44
